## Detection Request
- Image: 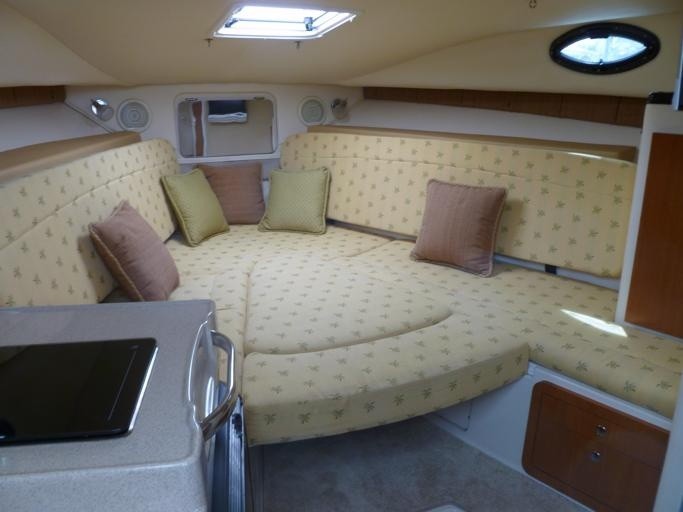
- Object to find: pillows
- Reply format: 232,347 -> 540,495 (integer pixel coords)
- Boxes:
258,167 -> 331,235
196,161 -> 266,224
160,169 -> 229,247
409,178 -> 507,277
88,199 -> 179,302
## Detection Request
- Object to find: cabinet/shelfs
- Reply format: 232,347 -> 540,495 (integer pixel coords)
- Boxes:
521,381 -> 669,511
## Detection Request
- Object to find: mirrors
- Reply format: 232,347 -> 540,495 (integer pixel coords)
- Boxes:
173,91 -> 277,164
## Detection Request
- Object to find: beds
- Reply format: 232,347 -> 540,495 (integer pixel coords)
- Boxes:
1,125 -> 682,448
